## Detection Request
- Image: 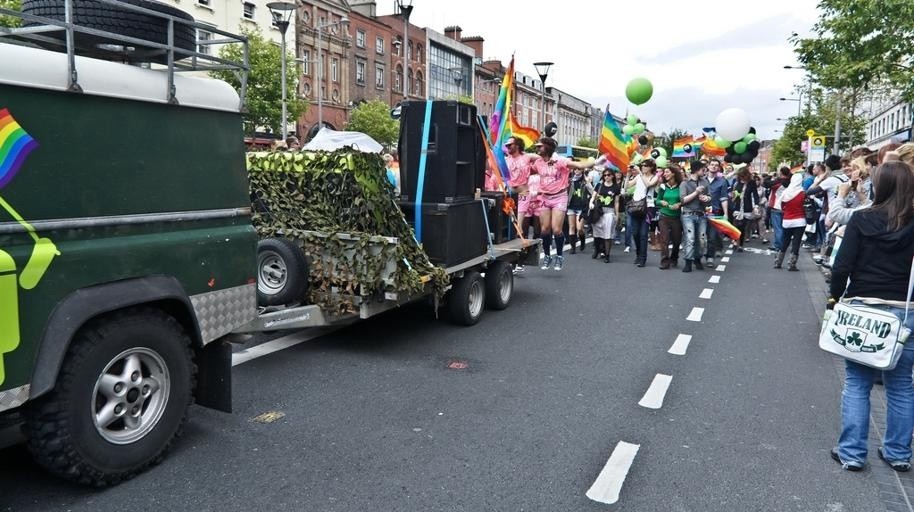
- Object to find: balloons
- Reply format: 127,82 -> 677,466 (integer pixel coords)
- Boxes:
627,113 -> 637,125
634,123 -> 645,134
715,114 -> 760,164
623,124 -> 633,136
626,77 -> 653,105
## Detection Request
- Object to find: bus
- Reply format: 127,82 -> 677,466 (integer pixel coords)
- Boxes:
555,144 -> 599,163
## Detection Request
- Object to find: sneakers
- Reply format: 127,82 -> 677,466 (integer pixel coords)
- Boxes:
511,264 -> 527,274
720,231 -> 775,250
803,230 -> 833,291
540,254 -> 552,270
614,240 -> 620,246
591,247 -> 599,259
553,255 -> 567,272
705,256 -> 715,268
670,253 -> 679,267
878,446 -> 911,472
658,256 -> 671,270
681,259 -> 693,272
601,252 -> 609,263
632,255 -> 647,268
623,245 -> 630,253
830,446 -> 864,471
693,257 -> 704,270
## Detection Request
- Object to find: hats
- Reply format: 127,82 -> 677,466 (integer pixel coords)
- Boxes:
534,137 -> 558,148
826,154 -> 842,169
690,160 -> 708,172
504,137 -> 521,147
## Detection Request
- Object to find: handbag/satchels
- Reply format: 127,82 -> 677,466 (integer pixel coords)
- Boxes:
593,183 -> 603,205
626,199 -> 648,216
803,196 -> 816,225
819,301 -> 912,371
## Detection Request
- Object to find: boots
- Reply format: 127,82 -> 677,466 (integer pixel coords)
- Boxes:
772,251 -> 786,269
787,253 -> 800,271
568,235 -> 577,254
579,233 -> 586,251
650,231 -> 662,250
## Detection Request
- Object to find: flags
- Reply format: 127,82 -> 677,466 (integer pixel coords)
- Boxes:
490,57 -> 540,154
598,107 -> 628,175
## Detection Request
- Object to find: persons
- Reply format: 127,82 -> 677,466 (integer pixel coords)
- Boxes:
382,154 -> 397,196
287,136 -> 300,152
825,160 -> 914,474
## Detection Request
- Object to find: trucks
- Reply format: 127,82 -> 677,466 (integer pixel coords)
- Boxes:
0,0 -> 256,493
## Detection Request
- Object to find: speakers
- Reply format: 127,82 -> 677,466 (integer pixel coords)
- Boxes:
392,100 -> 519,266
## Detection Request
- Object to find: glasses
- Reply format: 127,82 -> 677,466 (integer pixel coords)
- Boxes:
640,164 -> 648,166
604,173 -> 611,177
574,166 -> 582,171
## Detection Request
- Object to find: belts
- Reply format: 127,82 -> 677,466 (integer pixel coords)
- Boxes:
682,210 -> 704,215
536,188 -> 567,194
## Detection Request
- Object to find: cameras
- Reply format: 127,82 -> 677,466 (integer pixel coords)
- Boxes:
851,180 -> 858,187
630,165 -> 634,170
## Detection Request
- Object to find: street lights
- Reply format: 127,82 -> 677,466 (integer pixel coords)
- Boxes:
317,17 -> 349,133
446,66 -> 463,100
477,75 -> 501,117
532,60 -> 554,140
772,57 -> 813,148
394,0 -> 413,104
266,0 -> 297,147
